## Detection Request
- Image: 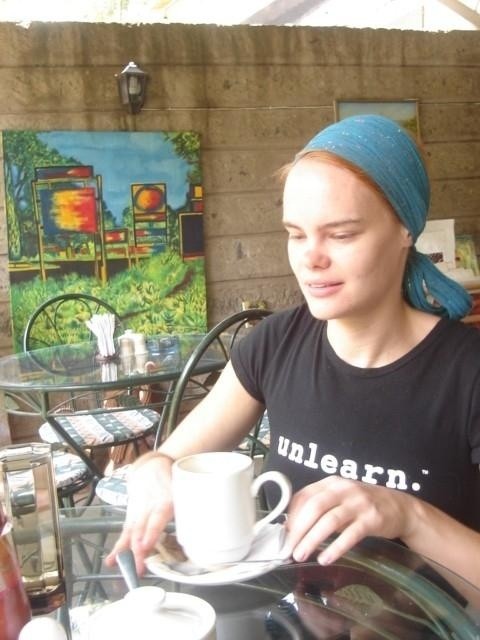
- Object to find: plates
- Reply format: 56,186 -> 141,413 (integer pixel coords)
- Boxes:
143,522 -> 293,586
76,588 -> 224,640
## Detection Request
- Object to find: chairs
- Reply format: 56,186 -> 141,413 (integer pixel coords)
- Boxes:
0,442 -> 110,602
90,309 -> 276,601
24,293 -> 162,517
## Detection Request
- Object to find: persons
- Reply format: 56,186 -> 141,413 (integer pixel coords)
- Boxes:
102,106 -> 479,639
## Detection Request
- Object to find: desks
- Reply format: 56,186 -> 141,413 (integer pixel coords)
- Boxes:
0,508 -> 480,640
0,334 -> 245,518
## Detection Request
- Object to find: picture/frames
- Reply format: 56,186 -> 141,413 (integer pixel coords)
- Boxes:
333,98 -> 422,151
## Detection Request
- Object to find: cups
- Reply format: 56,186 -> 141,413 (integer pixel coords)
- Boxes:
0,442 -> 64,596
168,450 -> 290,564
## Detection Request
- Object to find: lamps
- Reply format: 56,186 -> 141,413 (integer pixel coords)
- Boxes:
114,62 -> 150,115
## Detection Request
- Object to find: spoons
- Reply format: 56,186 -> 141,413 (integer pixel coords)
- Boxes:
160,558 -> 295,579
